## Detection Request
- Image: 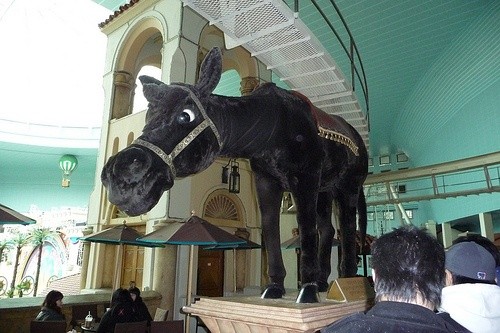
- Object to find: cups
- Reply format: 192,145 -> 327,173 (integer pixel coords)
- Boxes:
106,308 -> 110,311
84,315 -> 93,328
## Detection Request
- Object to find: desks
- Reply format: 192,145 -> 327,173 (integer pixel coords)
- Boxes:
81,323 -> 100,333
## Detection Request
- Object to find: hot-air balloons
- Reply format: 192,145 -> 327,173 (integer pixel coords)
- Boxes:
59,154 -> 78,188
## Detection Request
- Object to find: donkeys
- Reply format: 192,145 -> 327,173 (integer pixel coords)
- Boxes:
99,47 -> 370,304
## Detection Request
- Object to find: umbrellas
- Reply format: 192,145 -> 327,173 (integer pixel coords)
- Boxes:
81,219 -> 166,290
0,204 -> 36,225
137,209 -> 261,333
282,231 -> 341,249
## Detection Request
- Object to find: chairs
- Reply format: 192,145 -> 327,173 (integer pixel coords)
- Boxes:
30,320 -> 66,333
114,308 -> 185,333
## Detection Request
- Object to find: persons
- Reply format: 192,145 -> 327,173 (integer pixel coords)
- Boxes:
96,288 -> 145,333
316,224 -> 471,333
454,233 -> 500,286
34,290 -> 66,321
436,241 -> 500,333
128,287 -> 152,321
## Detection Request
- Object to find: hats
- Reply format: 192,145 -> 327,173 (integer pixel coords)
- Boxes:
445,241 -> 496,281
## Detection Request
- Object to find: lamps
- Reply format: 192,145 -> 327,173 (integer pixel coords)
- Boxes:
221,158 -> 240,193
